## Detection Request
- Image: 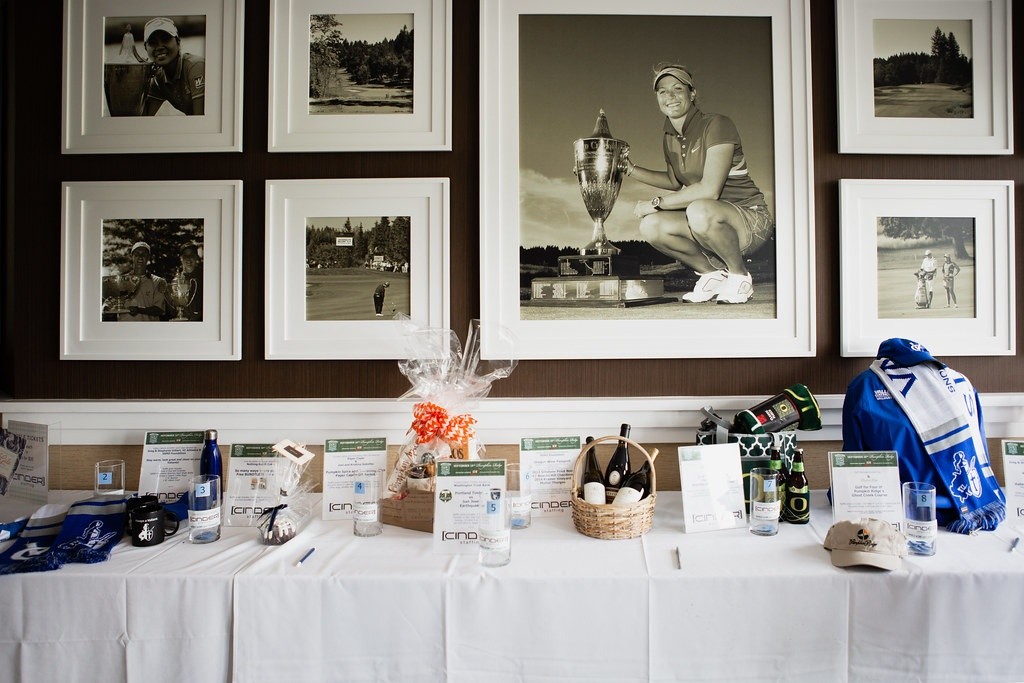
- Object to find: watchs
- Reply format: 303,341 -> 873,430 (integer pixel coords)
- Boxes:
651,196 -> 665,211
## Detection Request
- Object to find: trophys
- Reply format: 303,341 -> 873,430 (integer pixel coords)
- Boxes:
559,109 -> 640,278
164,268 -> 198,321
103,23 -> 160,117
102,268 -> 141,314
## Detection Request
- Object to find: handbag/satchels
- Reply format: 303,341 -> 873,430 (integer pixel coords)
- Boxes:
696,406 -> 797,514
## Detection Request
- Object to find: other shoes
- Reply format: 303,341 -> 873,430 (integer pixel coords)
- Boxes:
376,314 -> 383,317
944,306 -> 950,308
955,306 -> 958,308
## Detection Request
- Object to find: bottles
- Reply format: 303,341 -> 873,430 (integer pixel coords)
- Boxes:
787,449 -> 811,524
613,447 -> 659,506
198,430 -> 222,475
584,436 -> 606,505
766,446 -> 786,523
605,423 -> 630,502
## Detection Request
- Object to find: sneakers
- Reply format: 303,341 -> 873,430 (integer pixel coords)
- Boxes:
683,269 -> 729,303
716,272 -> 754,303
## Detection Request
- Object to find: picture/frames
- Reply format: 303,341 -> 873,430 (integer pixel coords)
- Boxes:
480,0 -> 818,360
61,0 -> 245,155
268,0 -> 453,152
839,178 -> 1016,357
836,0 -> 1016,155
60,179 -> 243,361
264,179 -> 450,361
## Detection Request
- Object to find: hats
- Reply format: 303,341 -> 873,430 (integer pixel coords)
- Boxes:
944,254 -> 950,257
925,250 -> 931,255
132,242 -> 150,252
180,242 -> 197,255
653,68 -> 693,90
823,516 -> 908,571
877,338 -> 946,368
144,18 -> 177,43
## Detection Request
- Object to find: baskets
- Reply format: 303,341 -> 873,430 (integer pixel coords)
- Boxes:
570,436 -> 656,540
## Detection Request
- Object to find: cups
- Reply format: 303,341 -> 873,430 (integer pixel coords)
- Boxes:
353,470 -> 382,536
902,482 -> 937,556
95,459 -> 126,495
189,475 -> 221,544
128,496 -> 179,547
507,463 -> 530,530
750,468 -> 779,536
480,493 -> 511,567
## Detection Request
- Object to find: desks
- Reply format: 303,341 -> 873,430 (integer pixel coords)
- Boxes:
0,490 -> 1024,683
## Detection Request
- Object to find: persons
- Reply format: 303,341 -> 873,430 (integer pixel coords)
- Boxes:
374,282 -> 390,317
102,242 -> 167,322
142,17 -> 206,117
627,65 -> 774,305
942,253 -> 960,308
920,249 -> 938,309
165,242 -> 203,322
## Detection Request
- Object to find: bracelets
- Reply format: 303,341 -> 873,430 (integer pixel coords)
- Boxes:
627,164 -> 636,177
